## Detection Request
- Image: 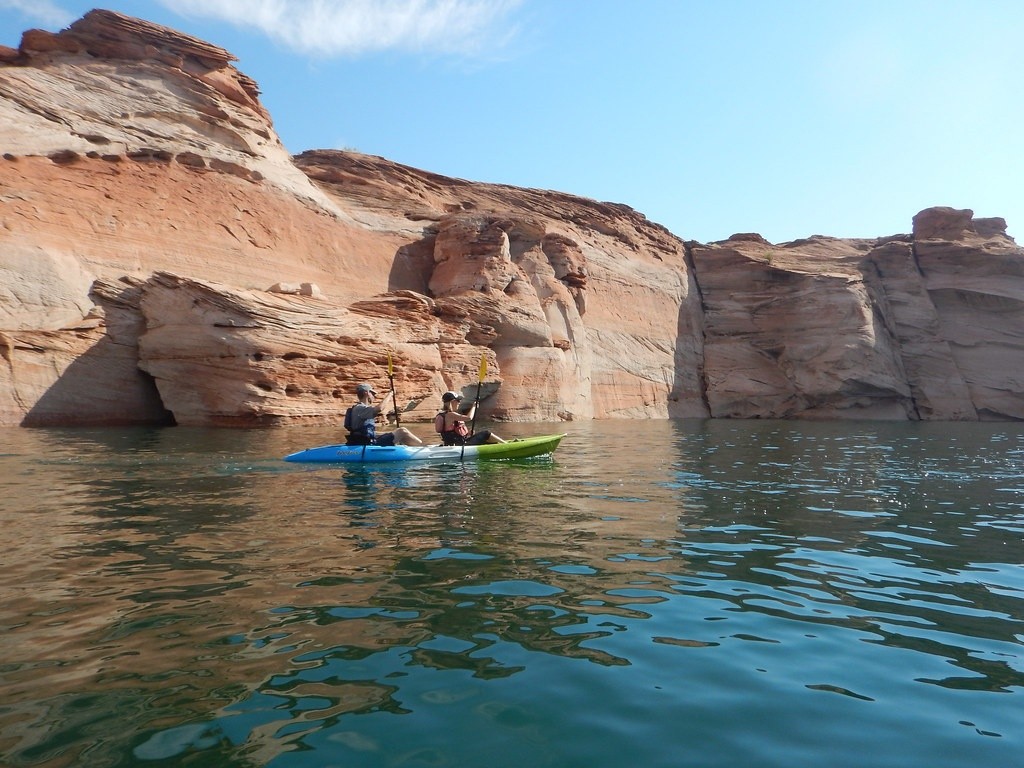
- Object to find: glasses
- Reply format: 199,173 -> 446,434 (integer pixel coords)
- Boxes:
455,400 -> 461,403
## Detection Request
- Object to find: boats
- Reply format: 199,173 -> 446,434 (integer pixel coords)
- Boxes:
283,434 -> 564,464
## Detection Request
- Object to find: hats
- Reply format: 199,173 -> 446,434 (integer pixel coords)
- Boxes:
356,384 -> 377,394
442,392 -> 464,402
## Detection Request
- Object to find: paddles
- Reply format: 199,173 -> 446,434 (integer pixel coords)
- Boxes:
470,353 -> 488,437
386,349 -> 403,446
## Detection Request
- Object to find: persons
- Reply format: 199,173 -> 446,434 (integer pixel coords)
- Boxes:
344,382 -> 427,446
436,391 -> 508,445
